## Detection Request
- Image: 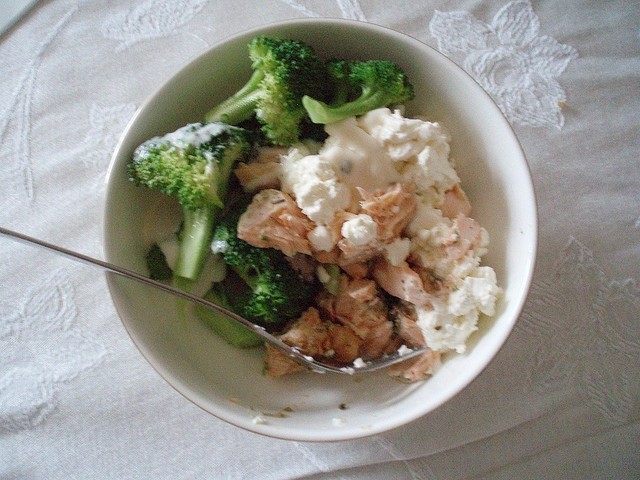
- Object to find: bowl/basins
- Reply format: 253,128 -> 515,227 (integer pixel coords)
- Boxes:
101,20 -> 538,441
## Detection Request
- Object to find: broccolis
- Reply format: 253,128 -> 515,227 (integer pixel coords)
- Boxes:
126,34 -> 416,349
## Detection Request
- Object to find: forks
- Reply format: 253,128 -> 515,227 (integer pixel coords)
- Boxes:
0,227 -> 429,377
